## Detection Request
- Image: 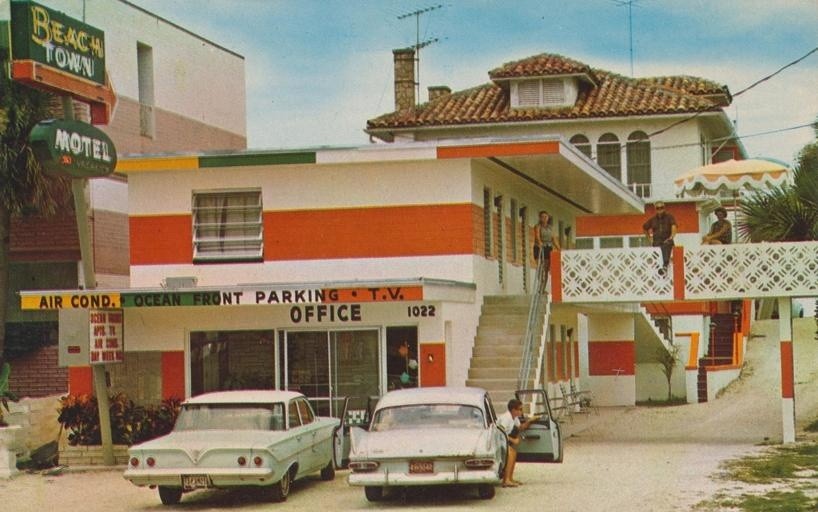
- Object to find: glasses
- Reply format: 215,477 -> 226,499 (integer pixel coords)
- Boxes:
656,206 -> 663,209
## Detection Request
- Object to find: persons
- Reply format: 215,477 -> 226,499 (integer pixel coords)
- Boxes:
495,397 -> 544,487
699,207 -> 734,245
531,209 -> 562,298
641,200 -> 680,271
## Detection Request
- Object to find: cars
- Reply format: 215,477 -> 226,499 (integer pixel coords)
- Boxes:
122,388 -> 342,507
331,385 -> 566,504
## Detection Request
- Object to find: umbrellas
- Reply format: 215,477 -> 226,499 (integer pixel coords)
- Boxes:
673,156 -> 792,242
